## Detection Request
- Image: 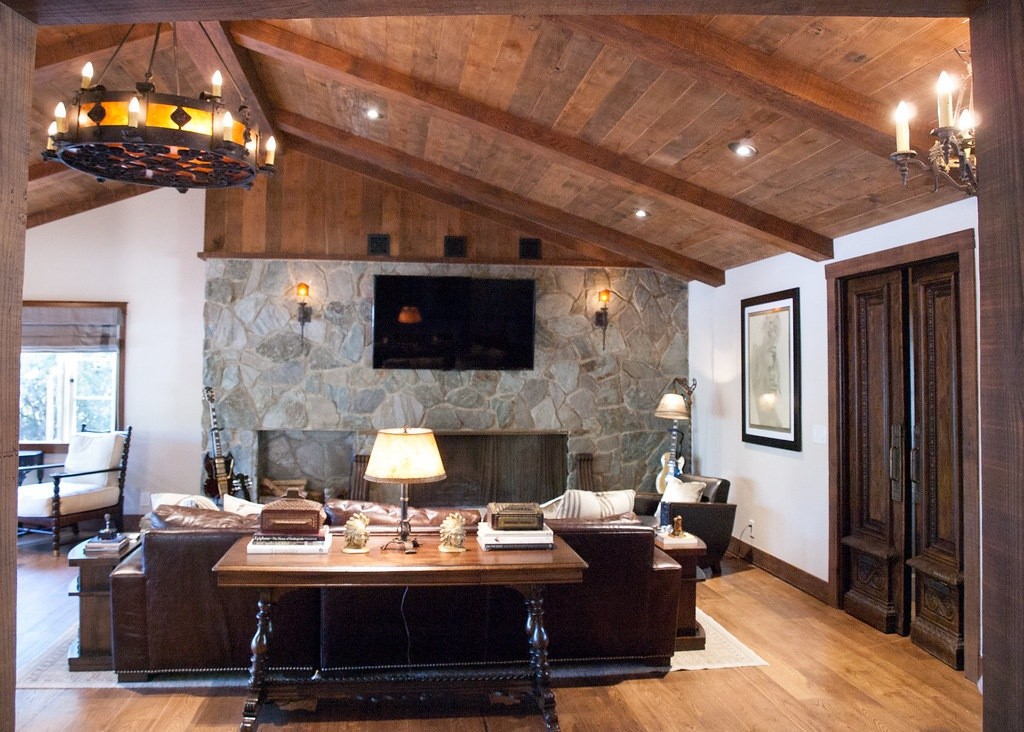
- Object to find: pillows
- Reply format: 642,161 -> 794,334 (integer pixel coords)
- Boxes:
149,492 -> 217,512
220,495 -> 265,516
556,487 -> 637,519
654,475 -> 707,520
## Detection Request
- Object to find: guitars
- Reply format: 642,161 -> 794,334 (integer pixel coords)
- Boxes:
200,384 -> 240,503
655,417 -> 686,495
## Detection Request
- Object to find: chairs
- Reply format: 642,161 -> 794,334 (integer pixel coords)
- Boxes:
17,423 -> 132,557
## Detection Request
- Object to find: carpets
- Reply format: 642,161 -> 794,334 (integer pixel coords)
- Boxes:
11,599 -> 769,690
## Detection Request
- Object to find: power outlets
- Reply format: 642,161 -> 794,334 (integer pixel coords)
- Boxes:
749,519 -> 755,538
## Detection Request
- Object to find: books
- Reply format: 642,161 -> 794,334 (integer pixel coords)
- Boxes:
247,526 -> 331,555
85,534 -> 129,551
476,522 -> 554,552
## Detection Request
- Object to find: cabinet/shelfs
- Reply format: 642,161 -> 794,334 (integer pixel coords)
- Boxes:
66,530 -> 142,669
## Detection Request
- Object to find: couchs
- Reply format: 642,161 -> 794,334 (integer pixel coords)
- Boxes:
111,509 -> 680,685
632,473 -> 739,573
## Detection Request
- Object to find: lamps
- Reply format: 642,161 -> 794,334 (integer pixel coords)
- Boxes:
655,391 -> 690,469
41,19 -> 279,193
362,425 -> 446,552
595,289 -> 611,327
297,282 -> 312,346
397,306 -> 423,324
888,44 -> 977,197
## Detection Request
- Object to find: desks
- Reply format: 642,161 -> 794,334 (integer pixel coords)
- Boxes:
209,536 -> 589,731
18,449 -> 44,485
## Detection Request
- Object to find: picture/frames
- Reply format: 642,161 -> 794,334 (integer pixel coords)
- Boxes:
740,287 -> 802,454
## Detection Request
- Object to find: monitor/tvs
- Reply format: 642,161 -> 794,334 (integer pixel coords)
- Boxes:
371,274 -> 535,371
